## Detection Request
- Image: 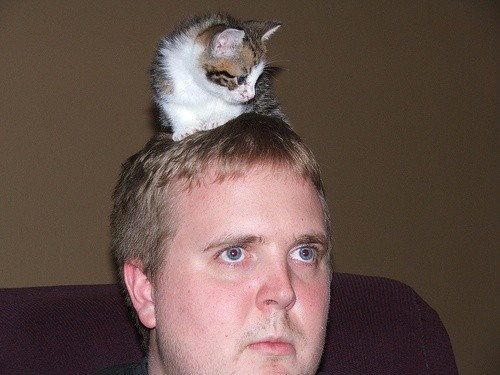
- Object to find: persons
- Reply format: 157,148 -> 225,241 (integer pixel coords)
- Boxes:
112,112 -> 333,375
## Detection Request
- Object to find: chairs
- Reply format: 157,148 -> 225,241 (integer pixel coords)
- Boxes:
0,273 -> 460,375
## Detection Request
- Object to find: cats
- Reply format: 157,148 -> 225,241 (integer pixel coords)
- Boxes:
149,10 -> 295,141
